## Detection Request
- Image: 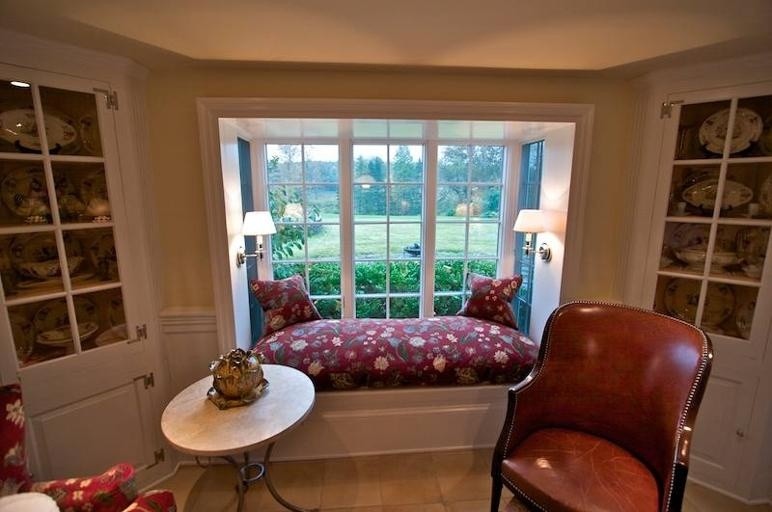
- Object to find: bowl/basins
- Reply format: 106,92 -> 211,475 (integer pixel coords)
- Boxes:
16,256 -> 85,281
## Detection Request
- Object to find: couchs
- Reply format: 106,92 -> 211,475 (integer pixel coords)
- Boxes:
0,384 -> 180,512
246,318 -> 517,463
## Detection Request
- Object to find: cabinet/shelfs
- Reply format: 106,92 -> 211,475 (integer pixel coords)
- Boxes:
653,81 -> 772,493
0,64 -> 176,497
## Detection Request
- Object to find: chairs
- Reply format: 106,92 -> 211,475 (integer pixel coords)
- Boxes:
490,299 -> 714,512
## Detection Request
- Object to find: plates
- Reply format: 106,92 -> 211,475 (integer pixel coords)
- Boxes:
16,271 -> 95,289
697,107 -> 762,155
683,179 -> 753,209
0,165 -> 68,217
33,297 -> 96,332
36,323 -> 99,355
8,230 -> 84,276
0,108 -> 77,151
735,226 -> 770,266
665,276 -> 734,325
89,232 -> 115,278
95,324 -> 126,347
759,173 -> 772,214
735,294 -> 758,341
8,312 -> 34,364
669,221 -> 732,262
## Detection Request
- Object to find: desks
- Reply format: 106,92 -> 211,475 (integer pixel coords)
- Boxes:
160,363 -> 320,512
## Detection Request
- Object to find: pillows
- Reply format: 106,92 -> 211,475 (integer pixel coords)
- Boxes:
249,273 -> 322,338
455,272 -> 522,331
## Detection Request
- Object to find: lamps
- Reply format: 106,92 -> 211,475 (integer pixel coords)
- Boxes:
236,211 -> 277,267
511,209 -> 551,263
